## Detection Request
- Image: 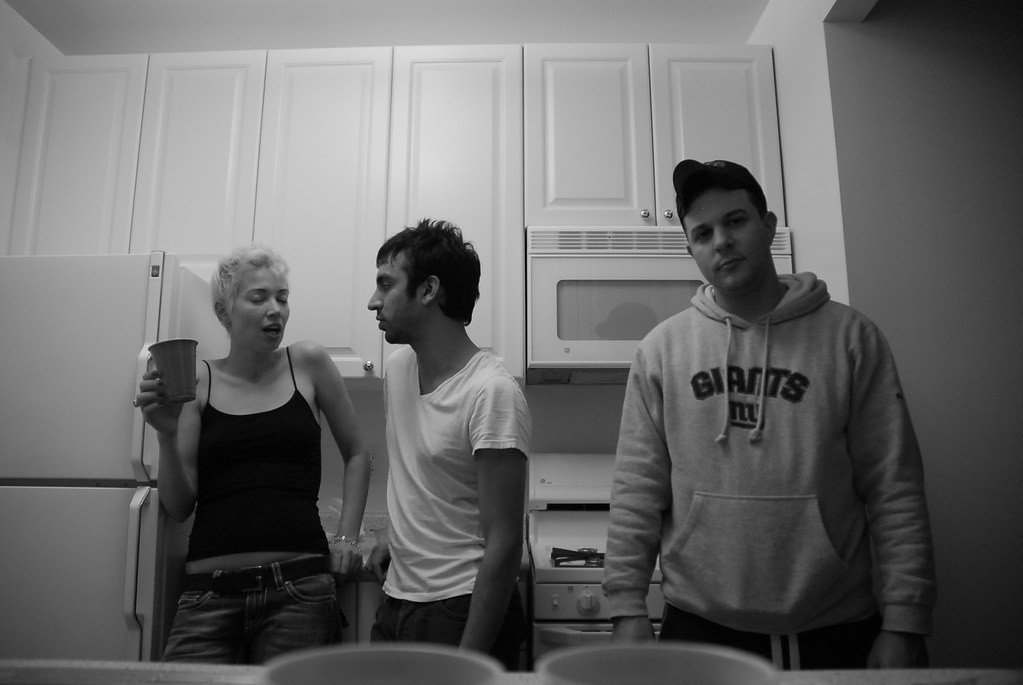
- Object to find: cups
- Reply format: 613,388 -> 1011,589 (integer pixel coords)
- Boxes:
147,338 -> 199,404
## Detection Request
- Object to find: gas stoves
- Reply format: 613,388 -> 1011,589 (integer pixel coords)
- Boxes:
528,453 -> 665,619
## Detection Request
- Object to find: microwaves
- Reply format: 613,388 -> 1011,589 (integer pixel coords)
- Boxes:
526,226 -> 793,384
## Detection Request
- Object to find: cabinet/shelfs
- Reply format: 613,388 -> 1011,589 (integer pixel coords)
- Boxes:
5,42 -> 792,393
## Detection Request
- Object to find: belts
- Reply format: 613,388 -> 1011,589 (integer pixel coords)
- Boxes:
185,557 -> 333,596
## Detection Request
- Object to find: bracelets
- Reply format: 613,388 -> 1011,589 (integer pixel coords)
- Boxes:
332,535 -> 361,552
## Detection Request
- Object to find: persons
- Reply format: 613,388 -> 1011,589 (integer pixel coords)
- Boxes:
599,159 -> 940,671
135,246 -> 373,665
358,218 -> 535,673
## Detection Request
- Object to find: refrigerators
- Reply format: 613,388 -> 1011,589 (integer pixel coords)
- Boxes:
0,251 -> 229,663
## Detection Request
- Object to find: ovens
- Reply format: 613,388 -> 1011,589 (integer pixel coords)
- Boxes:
531,623 -> 661,669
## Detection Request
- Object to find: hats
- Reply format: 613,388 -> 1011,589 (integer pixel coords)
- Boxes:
672,158 -> 766,222
